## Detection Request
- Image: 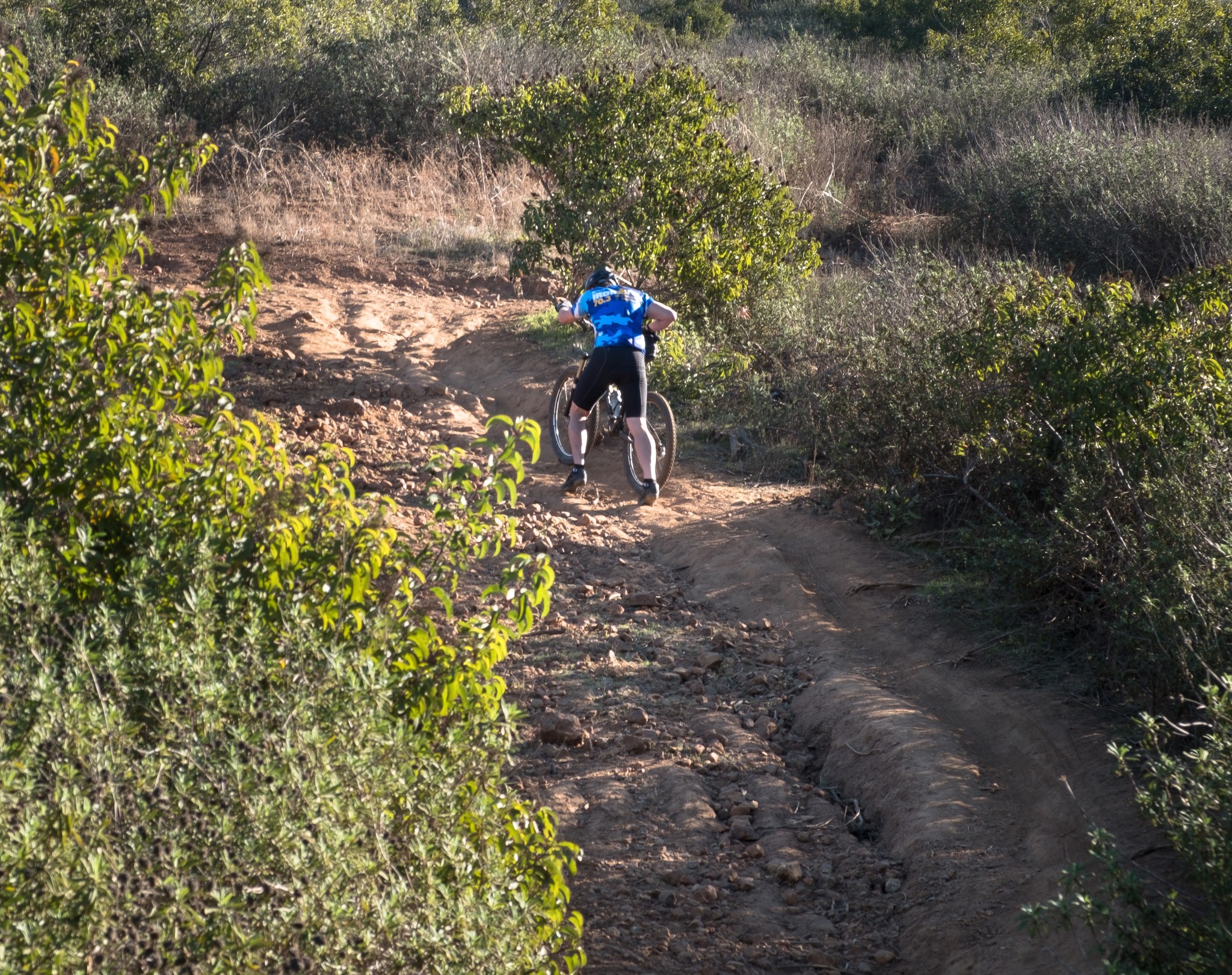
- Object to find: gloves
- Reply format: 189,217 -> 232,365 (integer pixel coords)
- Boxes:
556,302 -> 569,310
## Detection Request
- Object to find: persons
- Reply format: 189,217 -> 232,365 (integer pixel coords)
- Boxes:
557,266 -> 679,504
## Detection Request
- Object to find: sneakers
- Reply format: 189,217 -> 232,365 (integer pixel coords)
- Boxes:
637,481 -> 660,505
560,466 -> 587,492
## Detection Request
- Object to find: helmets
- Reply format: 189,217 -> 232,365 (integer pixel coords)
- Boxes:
585,269 -> 619,291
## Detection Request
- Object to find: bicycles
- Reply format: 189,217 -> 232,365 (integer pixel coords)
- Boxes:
547,297 -> 676,496
